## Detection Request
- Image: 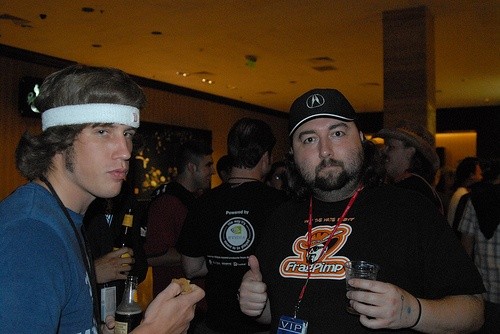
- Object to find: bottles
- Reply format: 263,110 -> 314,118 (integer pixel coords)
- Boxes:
112,207 -> 134,281
114,276 -> 142,334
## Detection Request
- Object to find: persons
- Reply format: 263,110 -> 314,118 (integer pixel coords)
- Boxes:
1,66 -> 205,334
239,87 -> 486,333
86,118 -> 499,334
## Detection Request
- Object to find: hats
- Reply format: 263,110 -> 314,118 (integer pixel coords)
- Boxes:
370,119 -> 436,166
287,89 -> 357,138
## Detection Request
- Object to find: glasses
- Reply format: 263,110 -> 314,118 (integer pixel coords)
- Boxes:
270,176 -> 282,184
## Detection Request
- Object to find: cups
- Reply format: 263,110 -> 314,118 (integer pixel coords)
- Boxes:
343,261 -> 380,316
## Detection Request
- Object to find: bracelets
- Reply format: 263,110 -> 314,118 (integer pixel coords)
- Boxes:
408,295 -> 422,329
